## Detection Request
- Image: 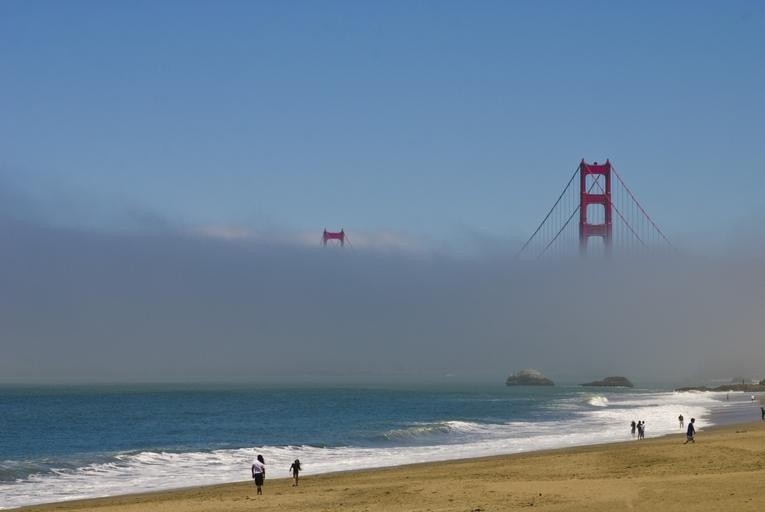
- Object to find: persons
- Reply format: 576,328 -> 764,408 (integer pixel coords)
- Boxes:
630,421 -> 645,440
679,415 -> 683,428
289,460 -> 302,486
761,408 -> 765,421
684,418 -> 696,444
751,394 -> 754,403
251,455 -> 265,495
